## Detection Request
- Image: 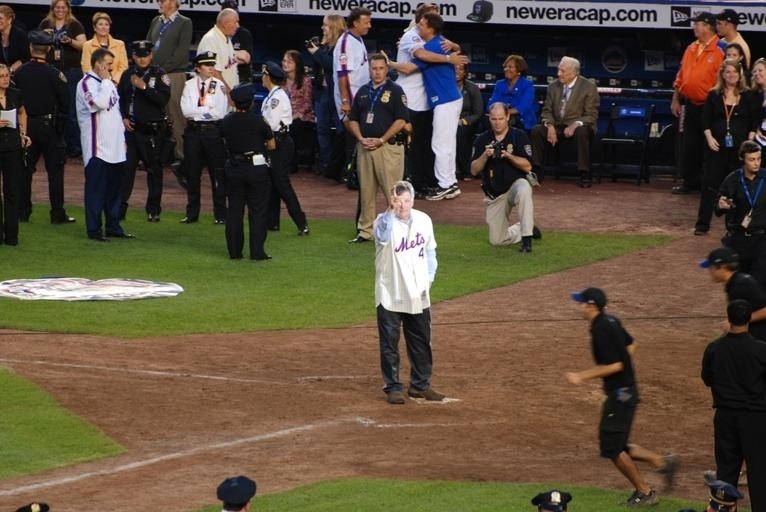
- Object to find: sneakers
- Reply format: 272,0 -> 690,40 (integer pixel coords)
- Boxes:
381,386 -> 407,405
616,488 -> 661,508
653,449 -> 682,495
424,183 -> 461,203
407,386 -> 448,403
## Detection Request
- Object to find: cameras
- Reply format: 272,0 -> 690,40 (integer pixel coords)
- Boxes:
52,40 -> 62,49
305,36 -> 320,47
493,142 -> 504,159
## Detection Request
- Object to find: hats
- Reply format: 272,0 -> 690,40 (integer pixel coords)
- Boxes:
696,246 -> 735,269
25,28 -> 54,46
213,472 -> 259,508
568,287 -> 608,309
685,6 -> 745,26
129,40 -> 155,59
706,480 -> 743,512
194,50 -> 289,109
464,1 -> 494,24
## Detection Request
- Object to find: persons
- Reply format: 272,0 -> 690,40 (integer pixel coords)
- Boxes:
306,4 -> 472,244
470,102 -> 542,254
216,475 -> 257,512
455,55 -> 599,189
669,8 -> 766,511
565,286 -> 676,507
370,180 -> 445,404
1,0 -> 315,261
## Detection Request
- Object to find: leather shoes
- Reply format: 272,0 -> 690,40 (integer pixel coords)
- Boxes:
515,175 -> 593,252
667,179 -> 711,238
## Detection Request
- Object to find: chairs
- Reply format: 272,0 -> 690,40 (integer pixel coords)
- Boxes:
542,100 -> 612,184
599,101 -> 658,187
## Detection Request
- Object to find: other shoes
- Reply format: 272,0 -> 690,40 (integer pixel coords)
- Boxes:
89,235 -> 109,244
214,219 -> 226,225
51,216 -> 77,225
106,231 -> 135,240
298,224 -> 309,236
3,237 -> 20,246
267,224 -> 279,230
17,216 -> 31,222
146,211 -> 160,222
262,254 -> 272,259
181,213 -> 199,224
